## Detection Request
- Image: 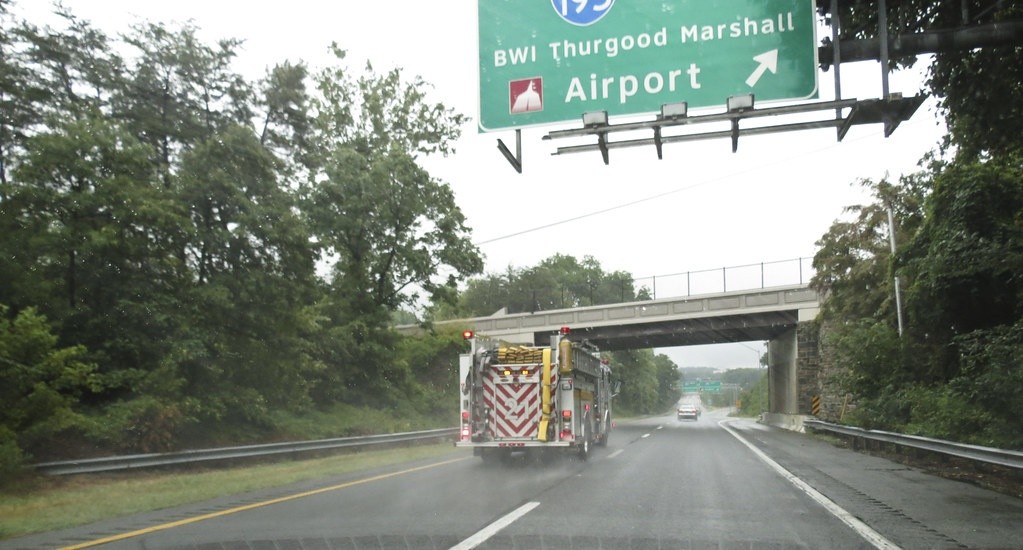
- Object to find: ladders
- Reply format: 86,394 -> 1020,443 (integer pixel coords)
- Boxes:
571,346 -> 602,379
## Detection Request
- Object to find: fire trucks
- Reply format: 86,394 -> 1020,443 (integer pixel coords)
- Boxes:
455,327 -> 620,463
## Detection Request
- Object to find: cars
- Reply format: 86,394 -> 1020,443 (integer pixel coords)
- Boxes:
678,404 -> 700,421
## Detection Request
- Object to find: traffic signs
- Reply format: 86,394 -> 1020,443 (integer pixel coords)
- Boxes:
700,379 -> 720,394
682,381 -> 699,393
477,0 -> 820,133
